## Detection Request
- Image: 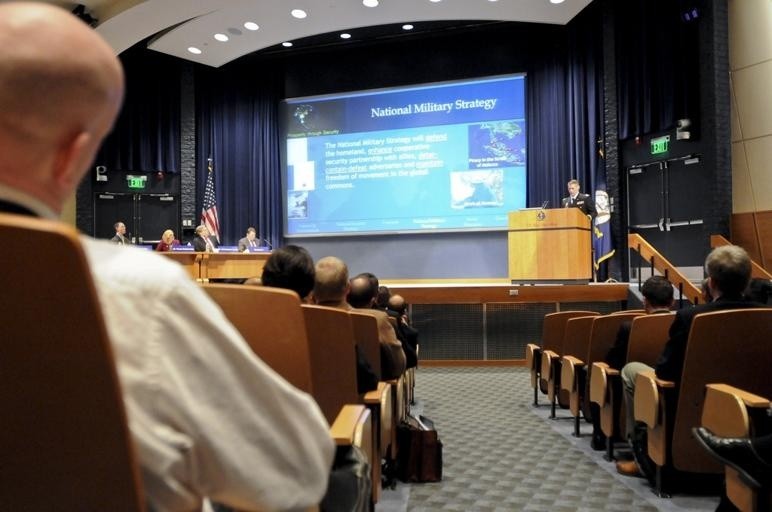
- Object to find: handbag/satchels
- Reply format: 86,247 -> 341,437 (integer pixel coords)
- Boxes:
396,412 -> 443,483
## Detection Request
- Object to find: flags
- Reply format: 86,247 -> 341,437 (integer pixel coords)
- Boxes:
591,147 -> 615,271
200,167 -> 220,245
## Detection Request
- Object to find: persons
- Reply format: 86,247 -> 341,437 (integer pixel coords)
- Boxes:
193,224 -> 220,252
700,278 -> 772,306
0,1 -> 338,512
245,245 -> 418,394
238,227 -> 260,253
562,180 -> 598,221
616,245 -> 751,479
110,222 -> 132,244
154,229 -> 180,252
591,276 -> 675,450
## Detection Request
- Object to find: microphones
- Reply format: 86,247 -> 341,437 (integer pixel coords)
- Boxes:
543,200 -> 549,209
542,200 -> 547,209
263,239 -> 272,246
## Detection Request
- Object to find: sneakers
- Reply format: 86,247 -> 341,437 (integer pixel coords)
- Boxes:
617,458 -> 642,477
